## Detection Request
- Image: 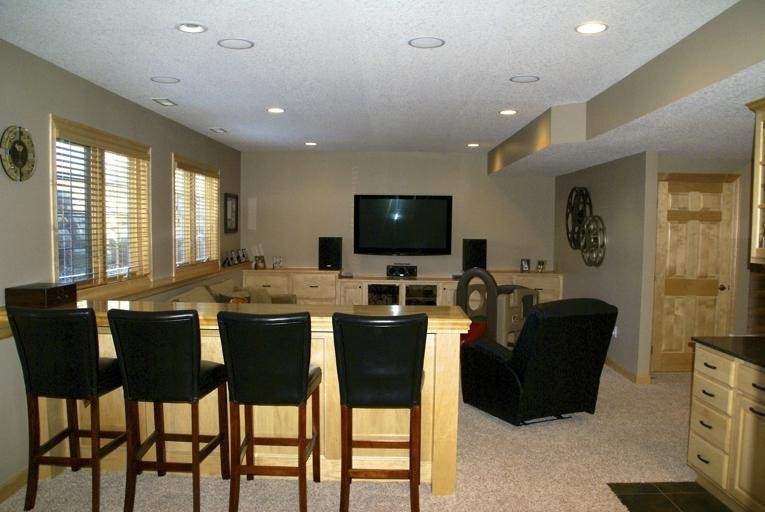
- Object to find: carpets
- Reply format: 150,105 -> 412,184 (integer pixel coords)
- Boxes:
606,481 -> 735,512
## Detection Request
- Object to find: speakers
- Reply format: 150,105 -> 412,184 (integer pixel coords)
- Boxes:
463,239 -> 487,272
319,237 -> 343,270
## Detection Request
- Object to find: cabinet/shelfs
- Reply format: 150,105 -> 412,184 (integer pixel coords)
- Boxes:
686,343 -> 764,512
750,103 -> 765,264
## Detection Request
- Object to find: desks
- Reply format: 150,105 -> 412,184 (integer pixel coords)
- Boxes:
242,268 -> 340,305
468,271 -> 563,311
338,276 -> 459,306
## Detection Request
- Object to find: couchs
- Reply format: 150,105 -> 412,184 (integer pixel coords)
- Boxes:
166,278 -> 297,302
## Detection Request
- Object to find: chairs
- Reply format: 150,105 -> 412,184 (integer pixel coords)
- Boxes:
217,311 -> 322,511
107,309 -> 230,512
460,298 -> 618,426
456,267 -> 497,342
332,313 -> 429,512
5,304 -> 127,512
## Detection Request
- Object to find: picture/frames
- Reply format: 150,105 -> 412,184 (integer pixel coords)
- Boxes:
223,191 -> 239,234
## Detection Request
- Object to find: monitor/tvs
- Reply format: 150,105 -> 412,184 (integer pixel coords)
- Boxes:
353,194 -> 453,256
